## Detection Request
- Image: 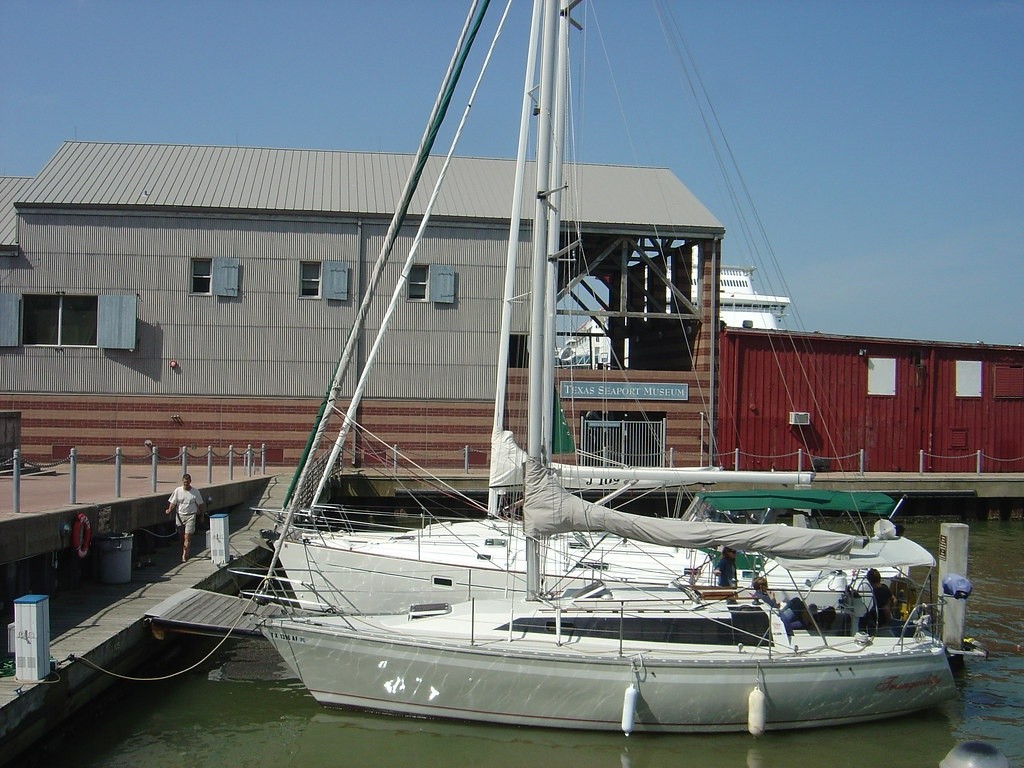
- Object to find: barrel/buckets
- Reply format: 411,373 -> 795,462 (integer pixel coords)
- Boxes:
96,534 -> 133,583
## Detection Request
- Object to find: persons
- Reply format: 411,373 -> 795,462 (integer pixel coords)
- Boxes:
752,577 -> 836,636
165,474 -> 204,562
845,569 -> 896,638
714,546 -> 739,587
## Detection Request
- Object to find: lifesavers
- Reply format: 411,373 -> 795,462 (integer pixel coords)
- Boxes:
70,511 -> 92,561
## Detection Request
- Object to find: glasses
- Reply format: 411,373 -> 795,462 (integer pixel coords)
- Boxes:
727,550 -> 738,554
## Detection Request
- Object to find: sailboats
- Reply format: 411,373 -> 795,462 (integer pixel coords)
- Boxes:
225,0 -> 959,738
